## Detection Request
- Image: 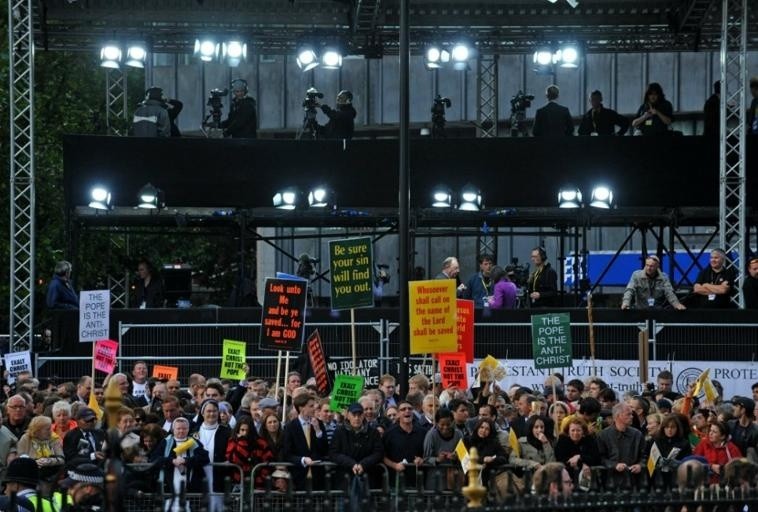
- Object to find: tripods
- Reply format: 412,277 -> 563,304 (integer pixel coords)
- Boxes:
510,112 -> 531,137
207,111 -> 225,138
296,111 -> 320,140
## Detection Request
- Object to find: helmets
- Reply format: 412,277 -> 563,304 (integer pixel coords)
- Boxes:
2,457 -> 40,486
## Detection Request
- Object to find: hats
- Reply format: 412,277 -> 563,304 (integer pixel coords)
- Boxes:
75,408 -> 97,422
57,463 -> 105,489
258,398 -> 280,409
730,397 -> 755,412
429,373 -> 442,384
347,403 -> 364,414
148,377 -> 169,389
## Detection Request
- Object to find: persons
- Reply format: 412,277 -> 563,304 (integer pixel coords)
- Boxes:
693,248 -> 733,309
1,363 -> 524,512
46,260 -> 79,309
743,259 -> 758,310
133,88 -> 171,139
631,82 -> 673,135
505,257 -> 520,273
131,261 -> 162,308
704,79 -> 737,136
146,89 -> 183,138
532,86 -> 575,137
527,246 -> 557,307
436,256 -> 464,298
510,370 -> 757,512
487,265 -> 517,310
311,90 -> 357,140
621,255 -> 687,311
747,76 -> 758,130
372,277 -> 384,302
462,253 -> 493,309
206,78 -> 257,139
578,90 -> 630,136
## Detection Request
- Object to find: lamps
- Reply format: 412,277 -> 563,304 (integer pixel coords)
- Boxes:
555,178 -> 614,209
294,39 -> 342,72
308,183 -> 329,208
530,38 -> 581,76
193,35 -> 248,67
428,175 -> 482,211
135,182 -> 155,209
87,181 -> 111,211
272,187 -> 297,210
98,38 -> 145,69
423,38 -> 473,72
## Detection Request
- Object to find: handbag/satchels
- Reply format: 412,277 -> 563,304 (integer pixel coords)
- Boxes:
350,472 -> 371,512
487,470 -> 525,505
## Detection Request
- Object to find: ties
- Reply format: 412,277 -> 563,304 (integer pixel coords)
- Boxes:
305,424 -> 312,479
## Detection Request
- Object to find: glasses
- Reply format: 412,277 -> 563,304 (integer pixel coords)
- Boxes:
399,407 -> 413,411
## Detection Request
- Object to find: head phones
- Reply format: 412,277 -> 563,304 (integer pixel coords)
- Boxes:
538,246 -> 546,261
345,90 -> 352,104
232,79 -> 249,93
